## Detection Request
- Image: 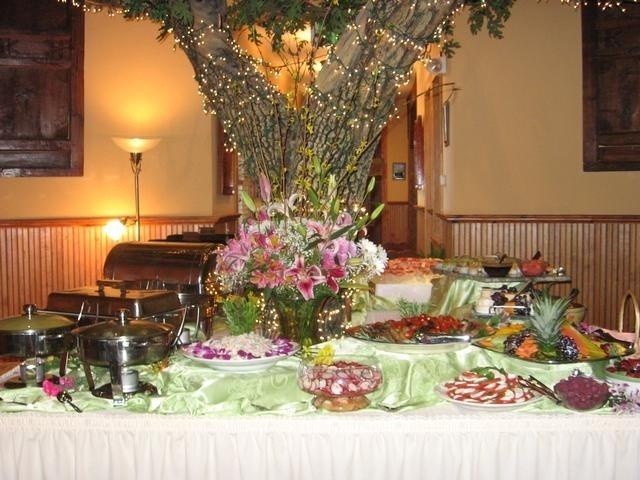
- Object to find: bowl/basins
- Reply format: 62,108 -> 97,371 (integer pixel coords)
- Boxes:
297,354 -> 384,411
604,363 -> 640,402
480,255 -> 514,277
558,302 -> 585,325
551,381 -> 610,412
516,259 -> 547,276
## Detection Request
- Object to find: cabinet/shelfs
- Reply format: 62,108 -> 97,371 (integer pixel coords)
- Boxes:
1,1 -> 84,177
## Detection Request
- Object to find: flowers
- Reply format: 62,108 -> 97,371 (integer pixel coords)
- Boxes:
186,24 -> 446,299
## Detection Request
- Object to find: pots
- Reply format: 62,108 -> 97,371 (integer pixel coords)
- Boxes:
0,304 -> 74,356
71,308 -> 173,366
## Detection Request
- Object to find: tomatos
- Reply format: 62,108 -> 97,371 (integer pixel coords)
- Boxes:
389,314 -> 463,340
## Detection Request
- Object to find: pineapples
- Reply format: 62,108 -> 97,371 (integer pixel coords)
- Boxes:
530,284 -> 575,361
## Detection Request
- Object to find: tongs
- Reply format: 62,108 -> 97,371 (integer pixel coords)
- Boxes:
518,374 -> 564,406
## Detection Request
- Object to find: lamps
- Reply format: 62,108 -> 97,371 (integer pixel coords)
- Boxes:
106,218 -> 137,236
111,138 -> 159,239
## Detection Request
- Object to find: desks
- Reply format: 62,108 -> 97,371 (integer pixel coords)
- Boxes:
48,288 -> 180,318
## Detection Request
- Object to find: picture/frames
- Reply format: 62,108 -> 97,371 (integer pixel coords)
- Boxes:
391,161 -> 408,180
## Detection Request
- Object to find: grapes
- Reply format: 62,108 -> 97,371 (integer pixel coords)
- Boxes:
502,329 -> 530,356
556,376 -> 608,409
514,295 -> 531,316
490,285 -> 518,306
556,335 -> 579,362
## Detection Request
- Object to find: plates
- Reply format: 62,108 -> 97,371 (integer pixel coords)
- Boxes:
340,325 -> 497,355
182,336 -> 300,374
434,374 -> 546,408
468,330 -> 636,367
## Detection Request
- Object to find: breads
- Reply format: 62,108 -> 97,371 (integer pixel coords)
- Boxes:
504,293 -> 532,302
504,302 -> 517,315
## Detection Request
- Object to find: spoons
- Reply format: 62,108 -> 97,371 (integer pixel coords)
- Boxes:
56,390 -> 82,413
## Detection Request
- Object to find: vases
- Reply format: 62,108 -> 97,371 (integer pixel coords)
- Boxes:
271,289 -> 325,346
